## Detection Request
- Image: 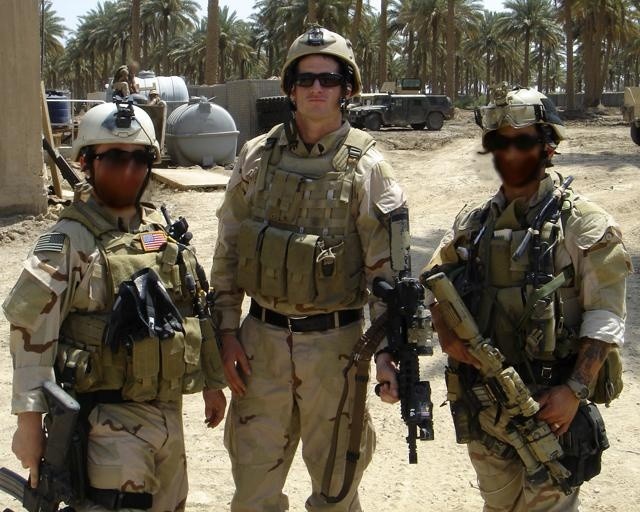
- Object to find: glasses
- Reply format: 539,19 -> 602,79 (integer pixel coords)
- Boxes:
92,148 -> 149,165
495,134 -> 542,150
294,73 -> 344,87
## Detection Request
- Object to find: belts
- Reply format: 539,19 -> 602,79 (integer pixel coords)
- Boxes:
249,298 -> 363,334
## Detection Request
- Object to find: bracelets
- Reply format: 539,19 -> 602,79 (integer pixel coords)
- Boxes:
373,348 -> 389,361
219,329 -> 237,336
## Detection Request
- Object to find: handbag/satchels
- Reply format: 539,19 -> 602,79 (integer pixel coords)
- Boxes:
560,403 -> 609,487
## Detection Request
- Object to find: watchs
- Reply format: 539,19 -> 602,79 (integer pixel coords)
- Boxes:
563,377 -> 591,401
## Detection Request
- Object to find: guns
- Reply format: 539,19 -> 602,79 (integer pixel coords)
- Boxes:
426,274 -> 573,494
366,275 -> 433,463
0,381 -> 152,512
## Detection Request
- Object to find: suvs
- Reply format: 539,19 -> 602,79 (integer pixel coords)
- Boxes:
622,85 -> 640,145
341,86 -> 457,134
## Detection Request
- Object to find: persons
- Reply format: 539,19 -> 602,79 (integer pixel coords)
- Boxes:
107,60 -> 166,109
419,88 -> 633,512
3,100 -> 228,512
207,26 -> 414,512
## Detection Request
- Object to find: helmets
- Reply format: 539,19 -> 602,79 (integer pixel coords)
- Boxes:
482,81 -> 567,149
281,24 -> 362,99
72,91 -> 161,163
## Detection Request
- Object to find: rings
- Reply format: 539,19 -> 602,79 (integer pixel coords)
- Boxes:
555,422 -> 561,429
